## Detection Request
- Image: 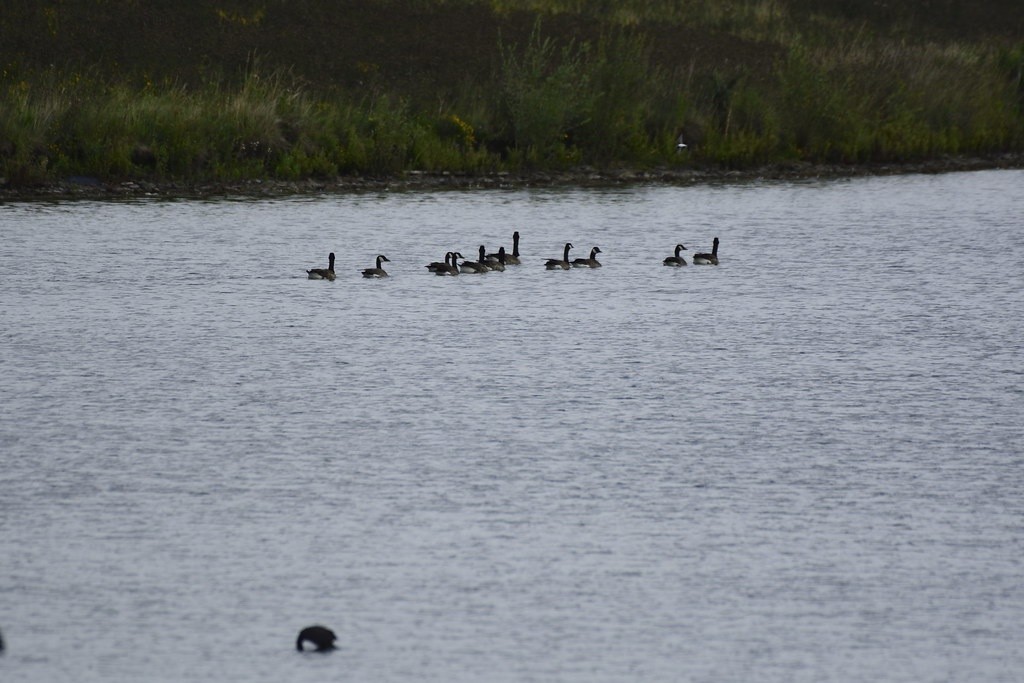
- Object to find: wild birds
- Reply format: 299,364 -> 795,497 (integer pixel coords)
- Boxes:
424,231 -> 522,276
295,623 -> 338,653
305,253 -> 336,282
692,237 -> 720,267
662,244 -> 688,267
361,254 -> 391,279
540,243 -> 603,271
